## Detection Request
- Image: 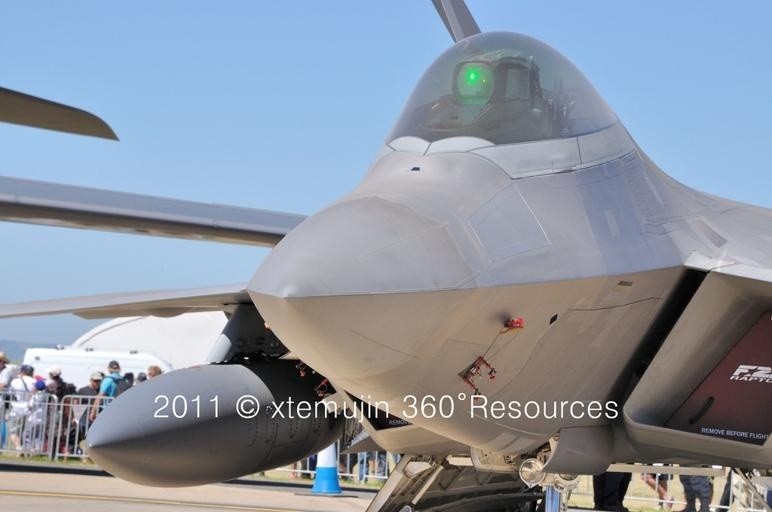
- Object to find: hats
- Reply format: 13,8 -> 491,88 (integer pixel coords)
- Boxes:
0,352 -> 10,364
109,360 -> 119,369
89,372 -> 103,381
21,364 -> 34,371
46,366 -> 62,376
32,381 -> 46,390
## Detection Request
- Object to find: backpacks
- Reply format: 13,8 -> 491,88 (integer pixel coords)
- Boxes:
105,375 -> 131,398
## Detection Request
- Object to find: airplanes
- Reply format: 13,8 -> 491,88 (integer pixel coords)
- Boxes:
0,0 -> 772,511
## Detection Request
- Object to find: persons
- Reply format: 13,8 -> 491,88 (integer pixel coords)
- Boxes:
290,424 -> 404,484
536,462 -> 772,512
0,351 -> 161,466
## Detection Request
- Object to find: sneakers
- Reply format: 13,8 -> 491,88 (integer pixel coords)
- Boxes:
664,497 -> 675,510
